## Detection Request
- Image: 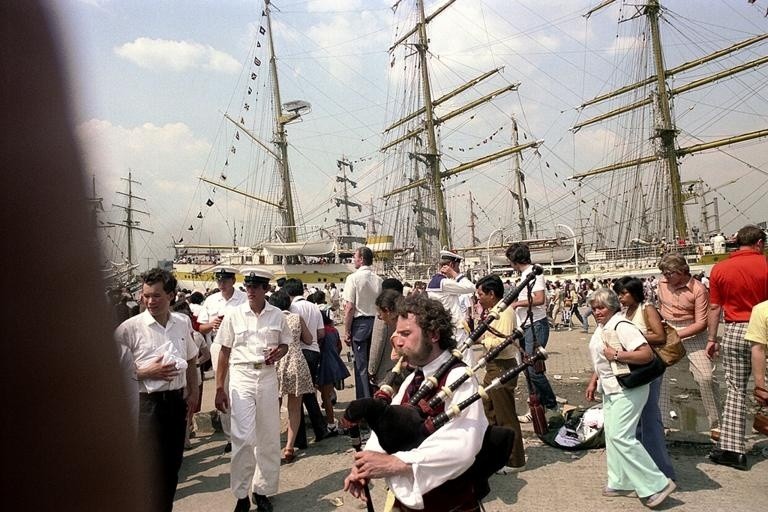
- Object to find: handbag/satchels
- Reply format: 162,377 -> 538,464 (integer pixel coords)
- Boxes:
644,322 -> 688,367
608,339 -> 669,391
751,411 -> 768,438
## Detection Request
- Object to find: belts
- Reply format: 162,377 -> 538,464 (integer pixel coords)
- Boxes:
353,315 -> 376,321
139,388 -> 185,400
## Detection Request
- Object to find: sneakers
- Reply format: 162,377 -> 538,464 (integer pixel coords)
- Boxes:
313,423 -> 337,443
496,464 -> 528,476
293,440 -> 308,449
709,428 -> 722,444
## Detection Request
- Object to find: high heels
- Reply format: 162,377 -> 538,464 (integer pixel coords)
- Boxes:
645,478 -> 678,508
283,446 -> 298,464
603,485 -> 639,499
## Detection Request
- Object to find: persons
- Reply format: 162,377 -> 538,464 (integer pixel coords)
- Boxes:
104,231 -> 768,510
2,0 -> 161,512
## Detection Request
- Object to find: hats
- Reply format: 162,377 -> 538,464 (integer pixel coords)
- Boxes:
239,265 -> 274,288
439,250 -> 465,263
211,266 -> 240,280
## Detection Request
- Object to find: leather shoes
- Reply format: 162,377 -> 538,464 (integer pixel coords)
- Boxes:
709,449 -> 750,472
223,443 -> 233,454
231,495 -> 252,512
250,492 -> 274,512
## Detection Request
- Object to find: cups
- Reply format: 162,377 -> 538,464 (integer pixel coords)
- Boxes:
217,315 -> 225,324
263,349 -> 274,365
